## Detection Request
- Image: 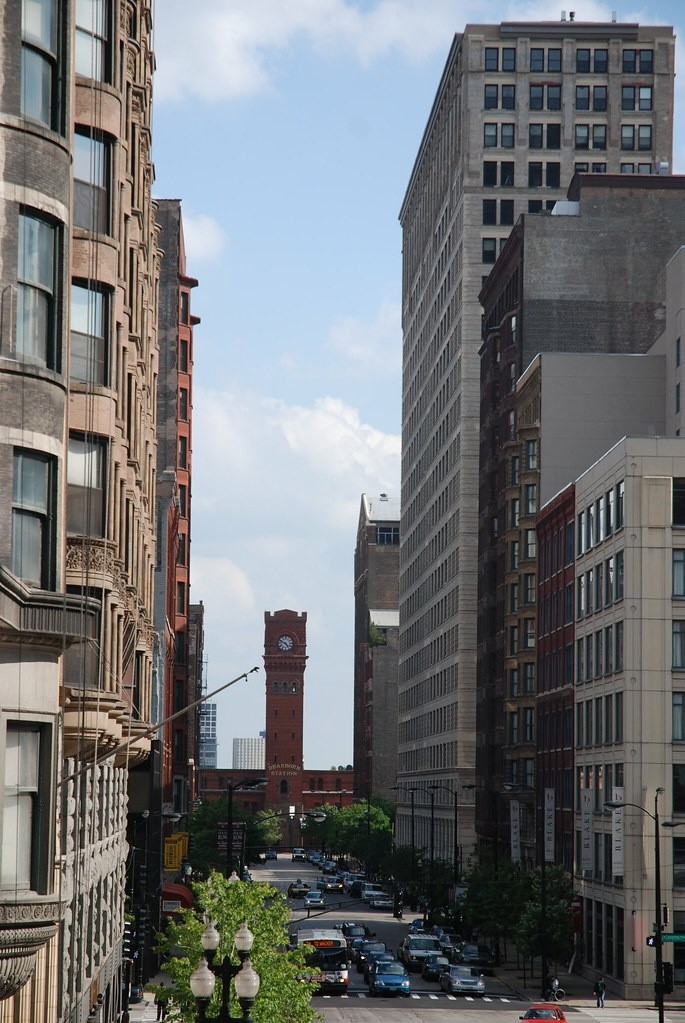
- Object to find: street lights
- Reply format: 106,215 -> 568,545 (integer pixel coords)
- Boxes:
427,786 -> 459,873
391,787 -> 435,913
225,777 -> 269,877
603,801 -> 665,1023
136,813 -> 182,983
503,782 -> 547,1001
462,785 -> 500,968
241,812 -> 329,882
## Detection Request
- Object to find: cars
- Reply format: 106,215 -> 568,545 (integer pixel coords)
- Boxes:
408,919 -> 495,973
519,1004 -> 566,1023
129,987 -> 142,1005
333,921 -> 394,984
422,955 -> 450,981
287,880 -> 311,899
304,892 -> 327,909
306,850 -> 392,910
397,938 -> 407,959
369,960 -> 413,999
440,964 -> 485,998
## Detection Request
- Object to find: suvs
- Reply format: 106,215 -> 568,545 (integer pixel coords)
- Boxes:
403,935 -> 443,970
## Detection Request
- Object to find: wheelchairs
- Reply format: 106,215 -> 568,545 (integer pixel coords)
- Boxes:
548,988 -> 566,1001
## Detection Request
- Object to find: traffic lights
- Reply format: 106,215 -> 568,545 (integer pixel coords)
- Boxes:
646,936 -> 656,947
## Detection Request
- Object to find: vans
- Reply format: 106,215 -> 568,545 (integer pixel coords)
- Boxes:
265,849 -> 277,860
291,848 -> 306,862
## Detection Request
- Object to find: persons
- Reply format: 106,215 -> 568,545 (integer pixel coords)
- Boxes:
543,976 -> 559,1001
593,977 -> 607,1008
154,982 -> 171,1022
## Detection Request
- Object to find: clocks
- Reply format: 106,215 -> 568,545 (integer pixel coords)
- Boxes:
277,635 -> 294,651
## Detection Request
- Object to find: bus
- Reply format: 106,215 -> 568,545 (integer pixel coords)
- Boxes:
296,930 -> 349,995
